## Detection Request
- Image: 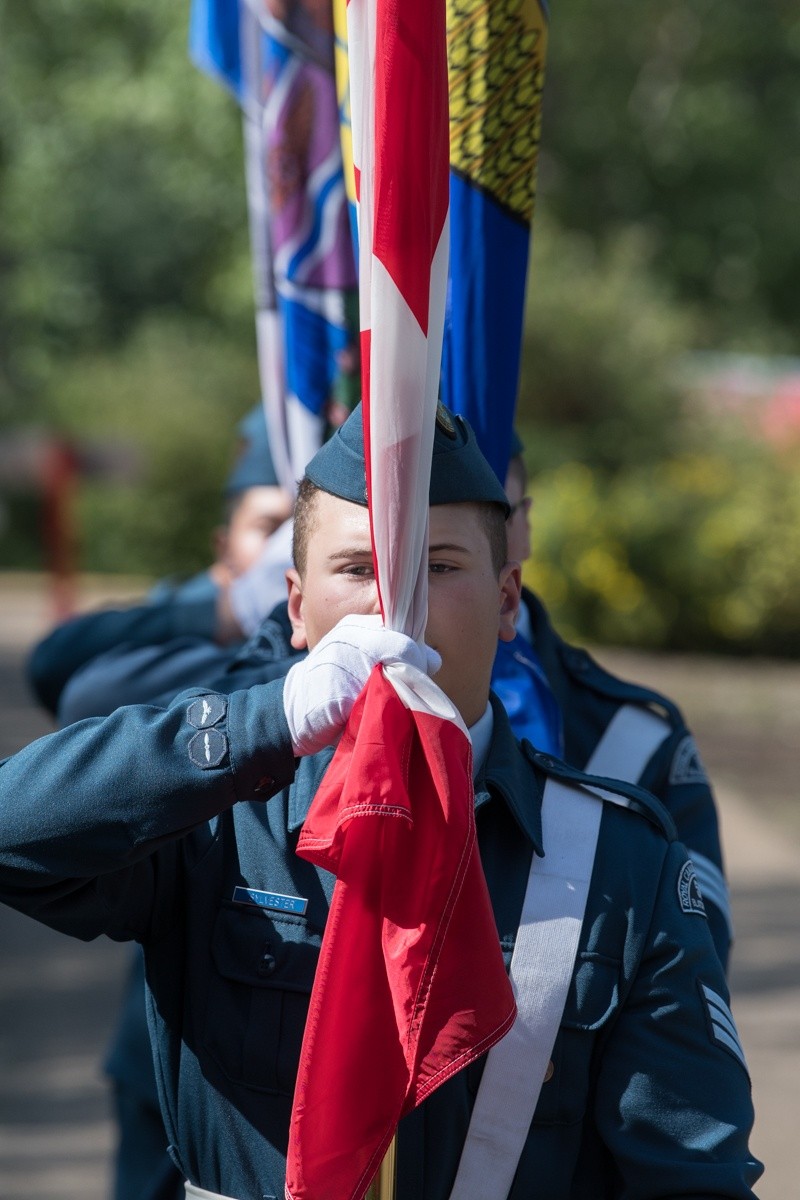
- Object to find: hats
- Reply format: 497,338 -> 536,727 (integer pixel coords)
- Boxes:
225,416 -> 281,488
305,398 -> 512,521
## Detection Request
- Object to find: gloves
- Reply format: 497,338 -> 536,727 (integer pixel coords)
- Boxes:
282,613 -> 443,758
226,514 -> 295,641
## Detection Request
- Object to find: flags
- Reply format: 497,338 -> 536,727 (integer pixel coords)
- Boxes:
196,0 -> 519,1200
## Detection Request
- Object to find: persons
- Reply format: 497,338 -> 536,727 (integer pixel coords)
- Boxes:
0,402 -> 725,1200
